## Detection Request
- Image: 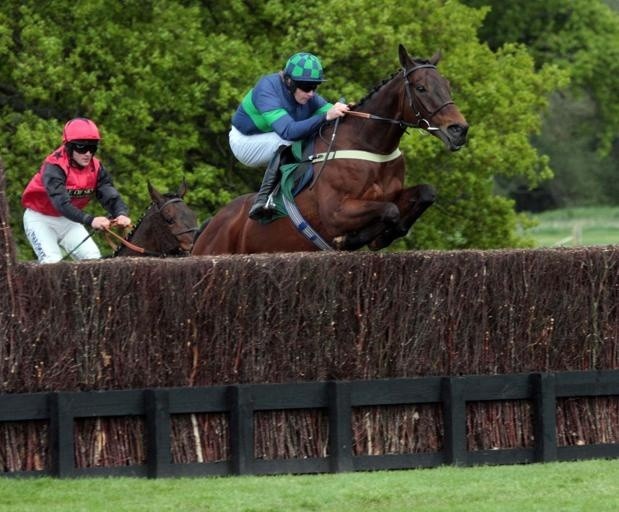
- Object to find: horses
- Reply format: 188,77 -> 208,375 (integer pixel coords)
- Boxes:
189,43 -> 470,257
98,175 -> 200,259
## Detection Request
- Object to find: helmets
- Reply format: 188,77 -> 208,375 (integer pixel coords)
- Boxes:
63,118 -> 100,144
283,52 -> 329,82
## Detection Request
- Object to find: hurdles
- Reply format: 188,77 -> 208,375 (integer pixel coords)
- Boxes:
0,172 -> 618,479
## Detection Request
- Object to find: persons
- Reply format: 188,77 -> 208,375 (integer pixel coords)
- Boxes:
229,53 -> 356,220
21,118 -> 131,265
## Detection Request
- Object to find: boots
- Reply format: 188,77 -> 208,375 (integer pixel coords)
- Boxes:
248,144 -> 296,220
